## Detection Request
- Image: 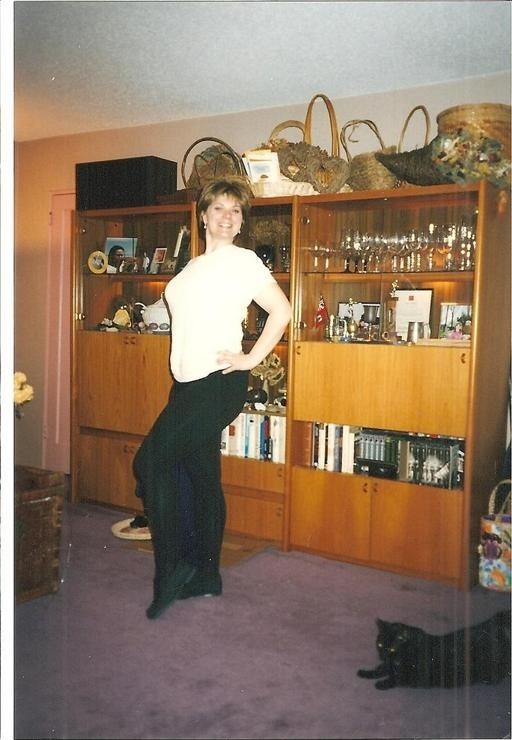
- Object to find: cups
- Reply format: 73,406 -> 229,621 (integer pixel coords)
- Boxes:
408,321 -> 430,345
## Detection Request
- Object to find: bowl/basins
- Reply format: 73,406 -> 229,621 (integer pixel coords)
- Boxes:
141,306 -> 170,332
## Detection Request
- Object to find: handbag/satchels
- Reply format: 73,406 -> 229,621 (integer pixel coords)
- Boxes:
479,513 -> 512,593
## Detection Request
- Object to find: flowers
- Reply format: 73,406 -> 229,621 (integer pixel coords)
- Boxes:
14,370 -> 34,420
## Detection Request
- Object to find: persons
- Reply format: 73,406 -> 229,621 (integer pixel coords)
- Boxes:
105,246 -> 131,274
133,176 -> 297,618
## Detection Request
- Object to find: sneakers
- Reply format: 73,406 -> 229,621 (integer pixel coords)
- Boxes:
144,557 -> 220,618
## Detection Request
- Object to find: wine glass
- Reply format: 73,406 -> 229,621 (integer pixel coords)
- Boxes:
308,226 -> 476,273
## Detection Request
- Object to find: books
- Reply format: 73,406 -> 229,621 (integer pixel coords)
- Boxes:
438,304 -> 473,337
218,413 -> 465,493
242,148 -> 280,184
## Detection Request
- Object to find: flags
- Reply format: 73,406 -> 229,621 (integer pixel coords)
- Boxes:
315,293 -> 329,329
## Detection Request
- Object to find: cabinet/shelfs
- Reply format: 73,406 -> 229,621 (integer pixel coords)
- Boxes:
14,464 -> 72,604
68,178 -> 511,593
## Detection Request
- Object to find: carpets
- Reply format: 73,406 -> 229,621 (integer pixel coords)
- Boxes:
121,534 -> 268,569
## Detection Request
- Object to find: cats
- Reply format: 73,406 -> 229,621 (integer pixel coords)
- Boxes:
356,607 -> 511,691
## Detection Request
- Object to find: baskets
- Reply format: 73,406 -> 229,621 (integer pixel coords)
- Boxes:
269,94 -> 351,193
340,119 -> 398,193
375,105 -> 465,186
152,102 -> 510,206
436,103 -> 511,162
156,137 -> 254,204
